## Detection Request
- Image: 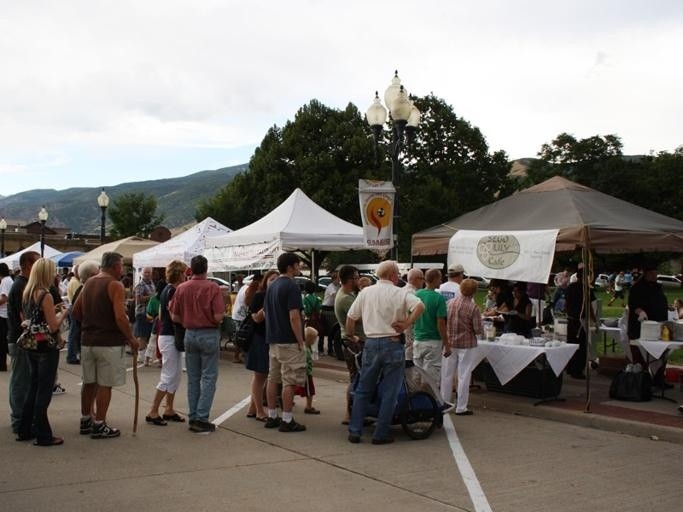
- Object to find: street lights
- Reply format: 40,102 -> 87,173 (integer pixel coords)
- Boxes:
97,187 -> 108,248
363,65 -> 420,260
36,203 -> 50,258
0,216 -> 8,257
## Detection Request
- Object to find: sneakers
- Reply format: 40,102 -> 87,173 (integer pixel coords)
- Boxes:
279,417 -> 306,432
90,421 -> 121,439
52,384 -> 66,394
265,417 -> 282,428
80,417 -> 95,435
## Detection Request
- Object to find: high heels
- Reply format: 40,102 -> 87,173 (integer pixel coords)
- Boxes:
163,414 -> 186,422
146,415 -> 168,426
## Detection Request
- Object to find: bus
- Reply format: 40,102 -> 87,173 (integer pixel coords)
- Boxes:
336,262 -> 445,281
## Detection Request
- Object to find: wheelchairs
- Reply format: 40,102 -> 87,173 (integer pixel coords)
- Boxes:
340,334 -> 456,442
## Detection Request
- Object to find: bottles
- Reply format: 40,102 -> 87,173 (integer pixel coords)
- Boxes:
661,326 -> 670,342
480,320 -> 497,342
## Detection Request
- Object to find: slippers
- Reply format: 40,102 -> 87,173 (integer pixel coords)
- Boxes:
16,436 -> 35,441
33,436 -> 64,446
246,414 -> 256,418
256,416 -> 269,422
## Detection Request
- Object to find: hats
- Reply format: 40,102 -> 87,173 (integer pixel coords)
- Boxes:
447,263 -> 464,273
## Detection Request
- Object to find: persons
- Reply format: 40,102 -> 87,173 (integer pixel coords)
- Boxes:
1,251 -> 483,446
484,259 -> 681,390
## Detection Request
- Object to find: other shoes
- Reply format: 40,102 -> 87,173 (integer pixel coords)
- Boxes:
456,410 -> 473,416
348,436 -> 361,444
188,419 -> 215,432
372,433 -> 395,446
304,407 -> 321,414
341,420 -> 351,425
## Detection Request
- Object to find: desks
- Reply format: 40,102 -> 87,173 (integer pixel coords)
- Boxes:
470,307 -> 682,406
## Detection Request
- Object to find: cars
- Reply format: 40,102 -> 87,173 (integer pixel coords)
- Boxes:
206,269 -> 378,299
463,273 -> 492,289
550,270 -> 683,291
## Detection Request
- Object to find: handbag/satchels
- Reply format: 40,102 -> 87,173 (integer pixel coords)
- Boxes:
609,371 -> 652,402
236,316 -> 255,351
15,322 -> 53,352
